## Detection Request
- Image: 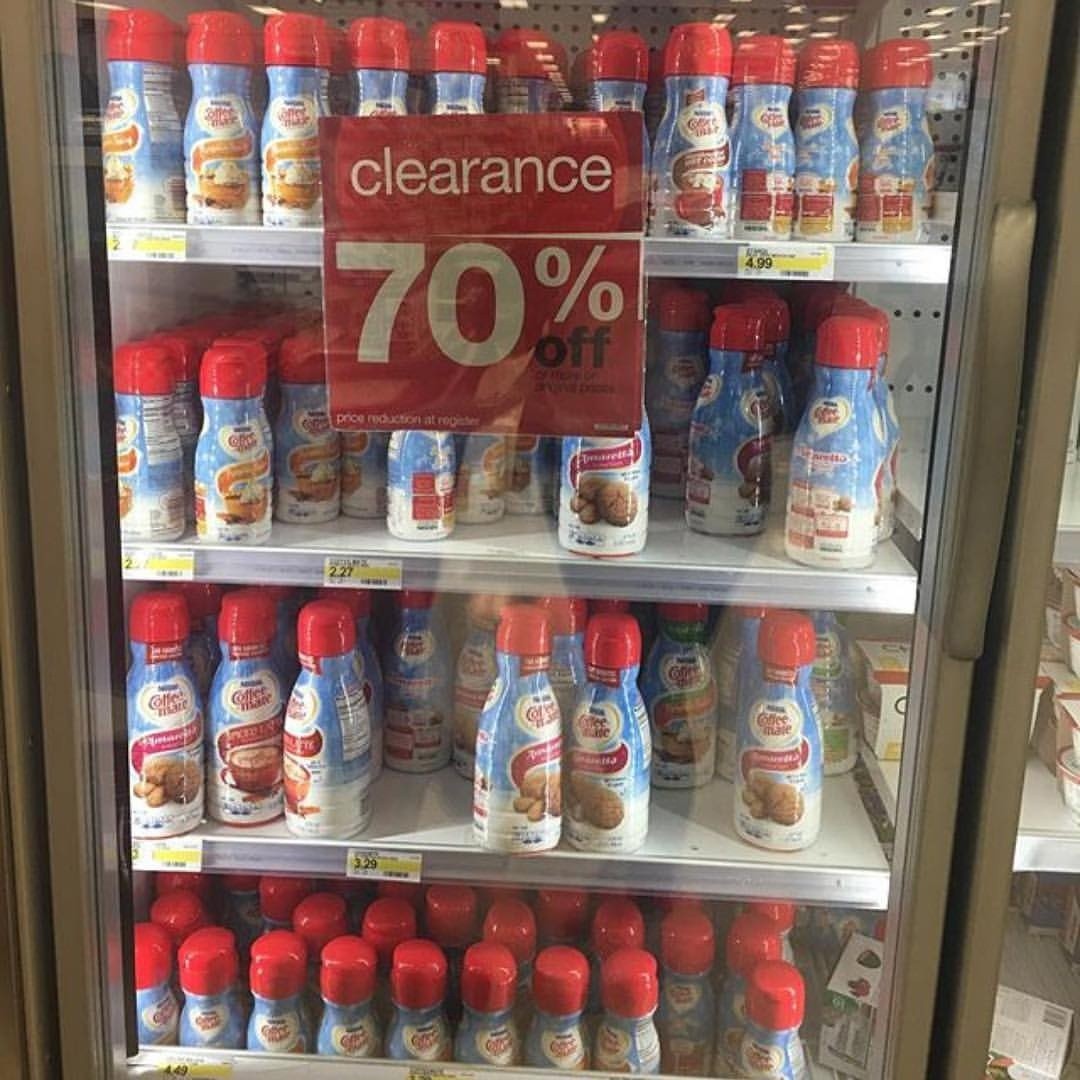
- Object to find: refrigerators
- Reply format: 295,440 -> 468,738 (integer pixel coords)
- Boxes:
0,1 -> 1078,1080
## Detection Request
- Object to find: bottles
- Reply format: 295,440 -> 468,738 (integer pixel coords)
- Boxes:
122,581 -> 853,853
98,9 -> 935,246
112,279 -> 901,570
132,868 -> 810,1080
